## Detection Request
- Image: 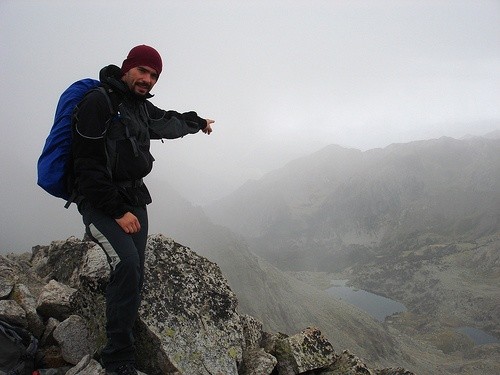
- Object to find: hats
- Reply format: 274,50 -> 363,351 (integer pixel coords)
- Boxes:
121,44 -> 162,75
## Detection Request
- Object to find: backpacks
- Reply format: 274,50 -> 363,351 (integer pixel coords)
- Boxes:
37,78 -> 114,209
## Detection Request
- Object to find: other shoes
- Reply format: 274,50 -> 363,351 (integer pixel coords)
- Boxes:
104,363 -> 139,375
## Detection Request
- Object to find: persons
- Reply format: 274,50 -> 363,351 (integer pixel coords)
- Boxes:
65,44 -> 215,374
